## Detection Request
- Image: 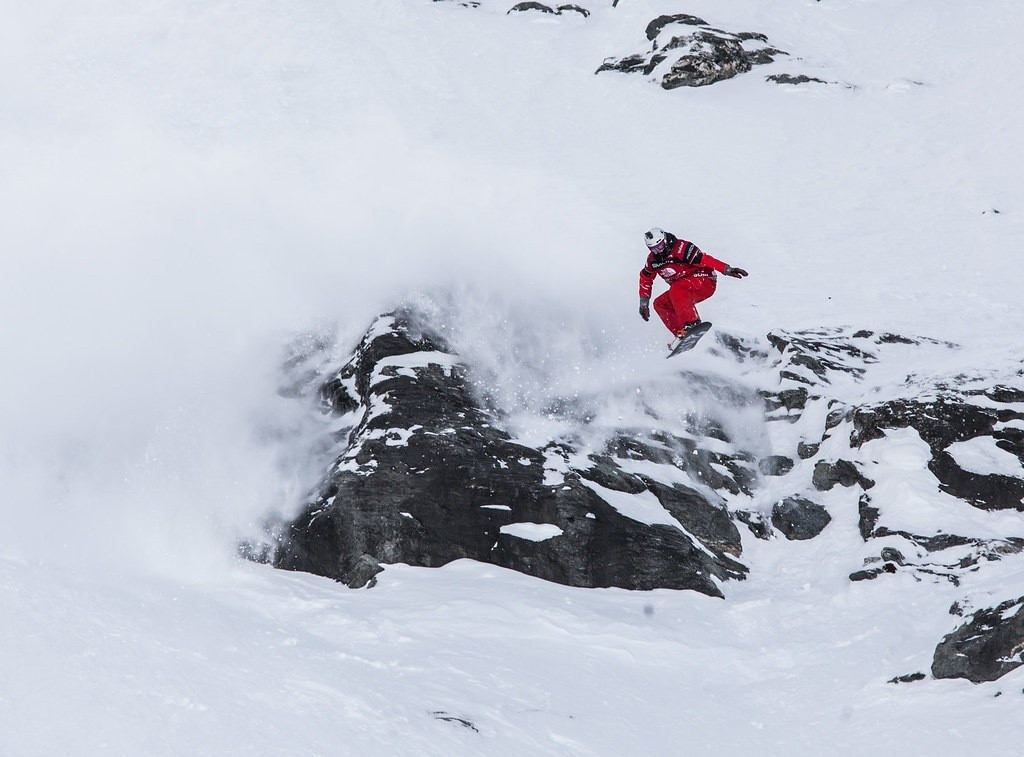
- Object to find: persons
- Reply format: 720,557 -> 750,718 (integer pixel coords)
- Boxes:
639,228 -> 748,343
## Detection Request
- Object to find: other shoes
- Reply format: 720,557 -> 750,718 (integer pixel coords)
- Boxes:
677,321 -> 701,338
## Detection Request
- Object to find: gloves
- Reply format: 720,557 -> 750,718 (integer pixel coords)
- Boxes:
724,267 -> 748,279
639,297 -> 650,322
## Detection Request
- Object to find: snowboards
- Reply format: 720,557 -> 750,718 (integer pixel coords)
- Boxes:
663,319 -> 713,363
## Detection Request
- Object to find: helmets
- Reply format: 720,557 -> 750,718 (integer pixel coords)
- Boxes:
645,227 -> 668,249
